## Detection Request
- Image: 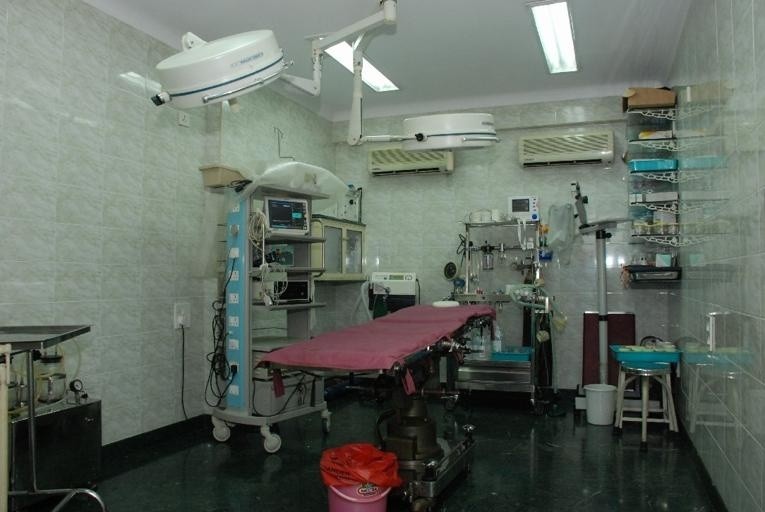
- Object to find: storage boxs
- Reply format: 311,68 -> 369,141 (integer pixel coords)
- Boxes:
493,344 -> 533,362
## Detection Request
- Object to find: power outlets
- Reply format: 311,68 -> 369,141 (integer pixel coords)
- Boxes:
174,302 -> 193,329
178,110 -> 190,128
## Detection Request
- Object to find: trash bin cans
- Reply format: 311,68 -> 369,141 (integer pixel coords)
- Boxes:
583,384 -> 618,425
320,444 -> 397,512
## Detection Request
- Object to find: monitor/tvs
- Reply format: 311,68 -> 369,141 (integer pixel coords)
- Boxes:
264,195 -> 310,235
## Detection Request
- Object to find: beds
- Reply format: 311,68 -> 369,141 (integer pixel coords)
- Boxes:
268,298 -> 496,390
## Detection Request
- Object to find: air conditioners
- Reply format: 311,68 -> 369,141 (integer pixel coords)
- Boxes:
366,147 -> 456,177
517,130 -> 615,166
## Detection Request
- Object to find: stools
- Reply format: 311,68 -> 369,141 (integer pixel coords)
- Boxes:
614,362 -> 681,450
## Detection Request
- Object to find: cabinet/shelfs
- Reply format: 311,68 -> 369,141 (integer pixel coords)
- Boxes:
310,216 -> 366,281
621,113 -> 684,283
249,234 -> 326,426
